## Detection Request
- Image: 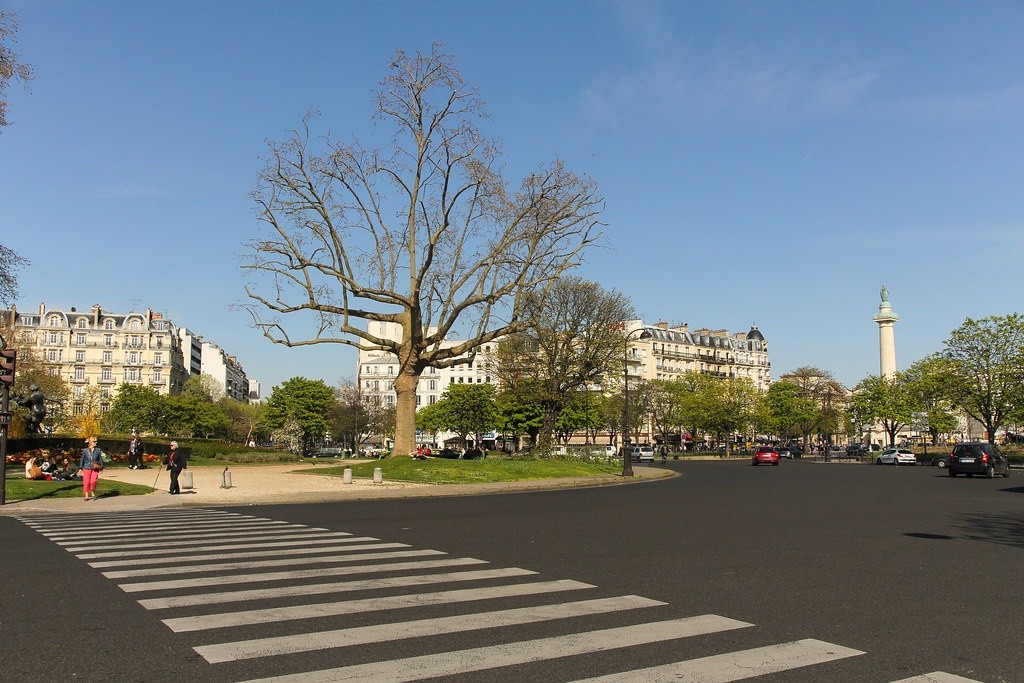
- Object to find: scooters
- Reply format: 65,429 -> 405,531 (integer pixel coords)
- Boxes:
662,450 -> 670,464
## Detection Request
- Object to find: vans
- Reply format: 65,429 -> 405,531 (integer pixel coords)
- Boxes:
630,446 -> 655,463
307,444 -> 352,458
511,444 -> 617,460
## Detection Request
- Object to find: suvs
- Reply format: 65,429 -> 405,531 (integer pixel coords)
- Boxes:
949,442 -> 1011,479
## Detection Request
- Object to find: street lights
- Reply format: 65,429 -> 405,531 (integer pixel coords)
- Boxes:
621,328 -> 653,476
929,397 -> 940,446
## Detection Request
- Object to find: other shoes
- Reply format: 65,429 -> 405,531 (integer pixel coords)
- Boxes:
84,498 -> 90,501
139,465 -> 144,469
168,491 -> 173,495
133,466 -> 137,470
127,467 -> 133,470
173,490 -> 180,494
92,496 -> 97,501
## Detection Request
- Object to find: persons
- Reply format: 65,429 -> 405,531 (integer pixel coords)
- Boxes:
162,441 -> 187,495
660,443 -> 670,464
25,430 -> 144,501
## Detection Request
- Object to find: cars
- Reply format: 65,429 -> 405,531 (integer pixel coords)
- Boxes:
752,446 -> 780,466
876,449 -> 917,466
845,443 -> 869,456
932,454 -> 951,468
780,447 -> 804,459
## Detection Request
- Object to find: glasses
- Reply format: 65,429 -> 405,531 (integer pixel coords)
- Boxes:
169,445 -> 174,446
93,441 -> 97,443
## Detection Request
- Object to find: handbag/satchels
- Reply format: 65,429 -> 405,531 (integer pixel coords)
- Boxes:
28,462 -> 42,479
92,461 -> 101,470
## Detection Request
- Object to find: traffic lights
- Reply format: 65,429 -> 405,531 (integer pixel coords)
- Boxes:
0,348 -> 16,387
